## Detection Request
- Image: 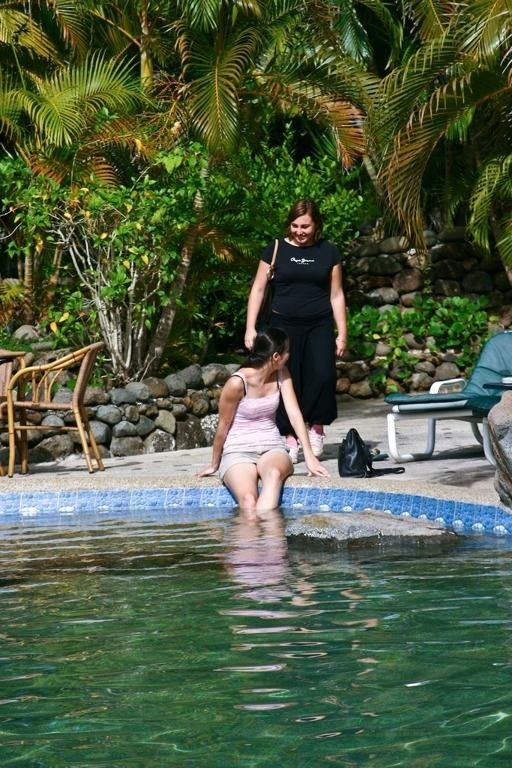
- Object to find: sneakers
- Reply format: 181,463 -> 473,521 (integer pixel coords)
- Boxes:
285,439 -> 302,464
308,430 -> 327,458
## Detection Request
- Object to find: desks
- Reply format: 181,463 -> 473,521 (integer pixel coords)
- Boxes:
1,348 -> 32,472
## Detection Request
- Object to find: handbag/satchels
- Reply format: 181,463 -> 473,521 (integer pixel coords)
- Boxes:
339,428 -> 372,478
249,270 -> 275,323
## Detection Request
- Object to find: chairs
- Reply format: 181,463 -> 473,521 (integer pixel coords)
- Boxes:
385,330 -> 511,463
6,342 -> 107,475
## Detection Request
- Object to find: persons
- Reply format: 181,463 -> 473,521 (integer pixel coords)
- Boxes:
223,509 -> 298,605
196,326 -> 330,509
244,199 -> 347,463
